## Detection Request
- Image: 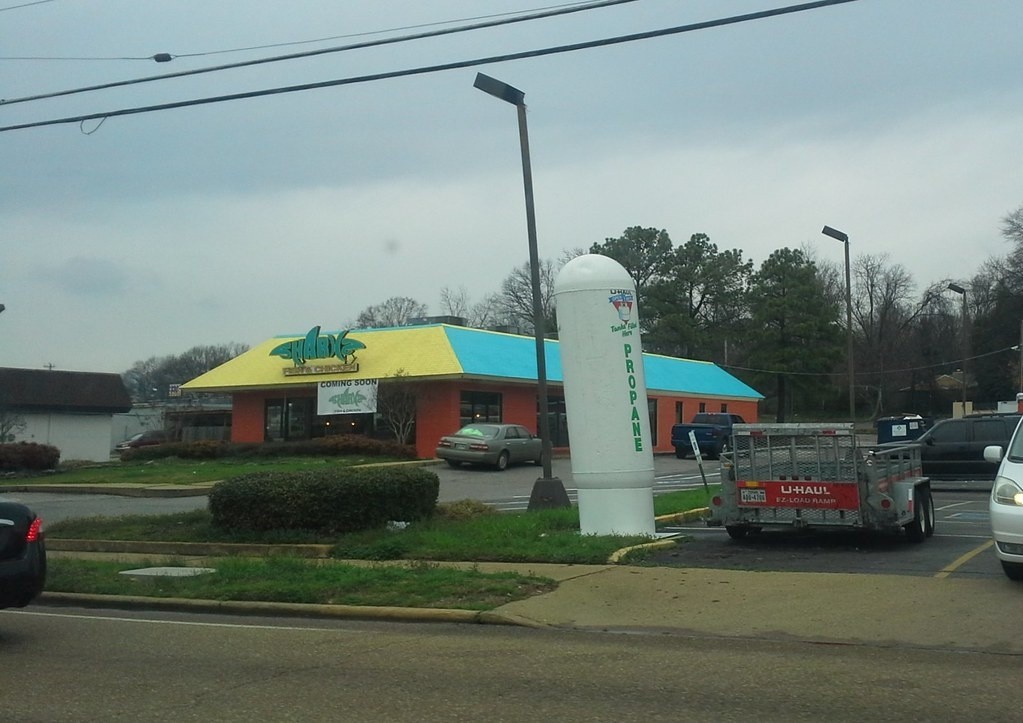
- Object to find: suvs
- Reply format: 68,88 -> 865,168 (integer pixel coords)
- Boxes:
870,411 -> 1023,481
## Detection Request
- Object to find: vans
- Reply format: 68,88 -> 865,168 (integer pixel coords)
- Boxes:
981,414 -> 1023,582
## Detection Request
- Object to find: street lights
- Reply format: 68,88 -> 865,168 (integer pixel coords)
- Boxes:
473,70 -> 574,513
822,224 -> 866,467
947,283 -> 967,416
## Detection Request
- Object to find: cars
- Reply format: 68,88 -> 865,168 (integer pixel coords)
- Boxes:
0,500 -> 48,613
434,420 -> 554,472
109,430 -> 175,462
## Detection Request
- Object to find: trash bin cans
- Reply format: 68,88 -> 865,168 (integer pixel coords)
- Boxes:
878,416 -> 929,443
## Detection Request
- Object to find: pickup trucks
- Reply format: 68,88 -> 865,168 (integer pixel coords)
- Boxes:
669,412 -> 763,460
